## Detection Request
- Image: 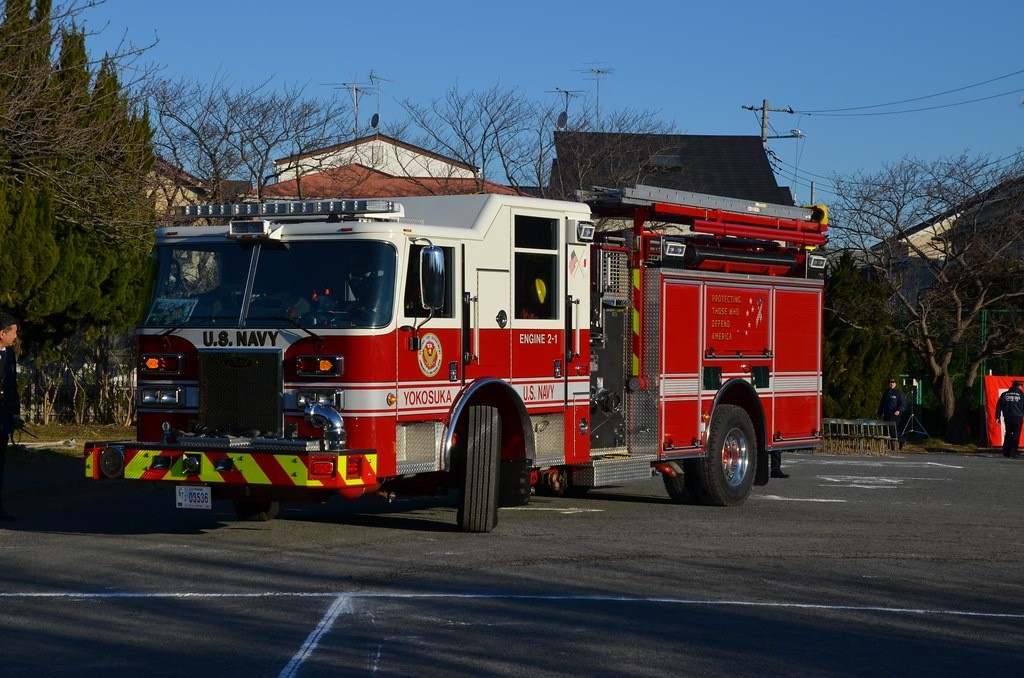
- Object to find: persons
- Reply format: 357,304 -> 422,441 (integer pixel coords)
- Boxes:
877,377 -> 907,450
0,312 -> 26,455
770,450 -> 790,479
996,380 -> 1024,458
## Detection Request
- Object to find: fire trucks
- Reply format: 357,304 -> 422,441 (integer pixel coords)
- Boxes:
84,184 -> 828,533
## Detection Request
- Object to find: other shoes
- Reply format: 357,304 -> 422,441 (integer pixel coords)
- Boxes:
1010,453 -> 1021,459
0,512 -> 16,521
899,440 -> 907,450
771,470 -> 789,478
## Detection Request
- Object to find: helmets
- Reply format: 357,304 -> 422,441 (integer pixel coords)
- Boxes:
888,378 -> 896,382
